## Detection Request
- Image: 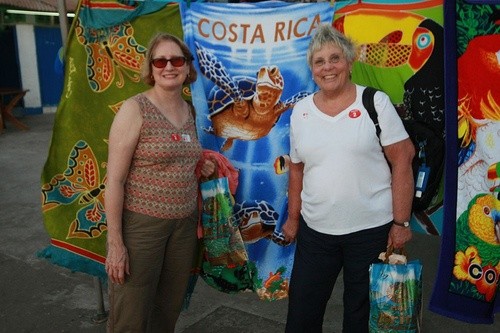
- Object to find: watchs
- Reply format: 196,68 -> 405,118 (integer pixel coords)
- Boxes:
393,219 -> 410,227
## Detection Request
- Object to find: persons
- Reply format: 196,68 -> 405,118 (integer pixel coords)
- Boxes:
105,33 -> 215,333
283,26 -> 415,333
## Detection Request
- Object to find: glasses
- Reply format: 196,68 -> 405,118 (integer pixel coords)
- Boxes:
151,56 -> 189,68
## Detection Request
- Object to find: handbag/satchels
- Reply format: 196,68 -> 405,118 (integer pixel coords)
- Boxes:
368,244 -> 423,333
198,160 -> 254,294
